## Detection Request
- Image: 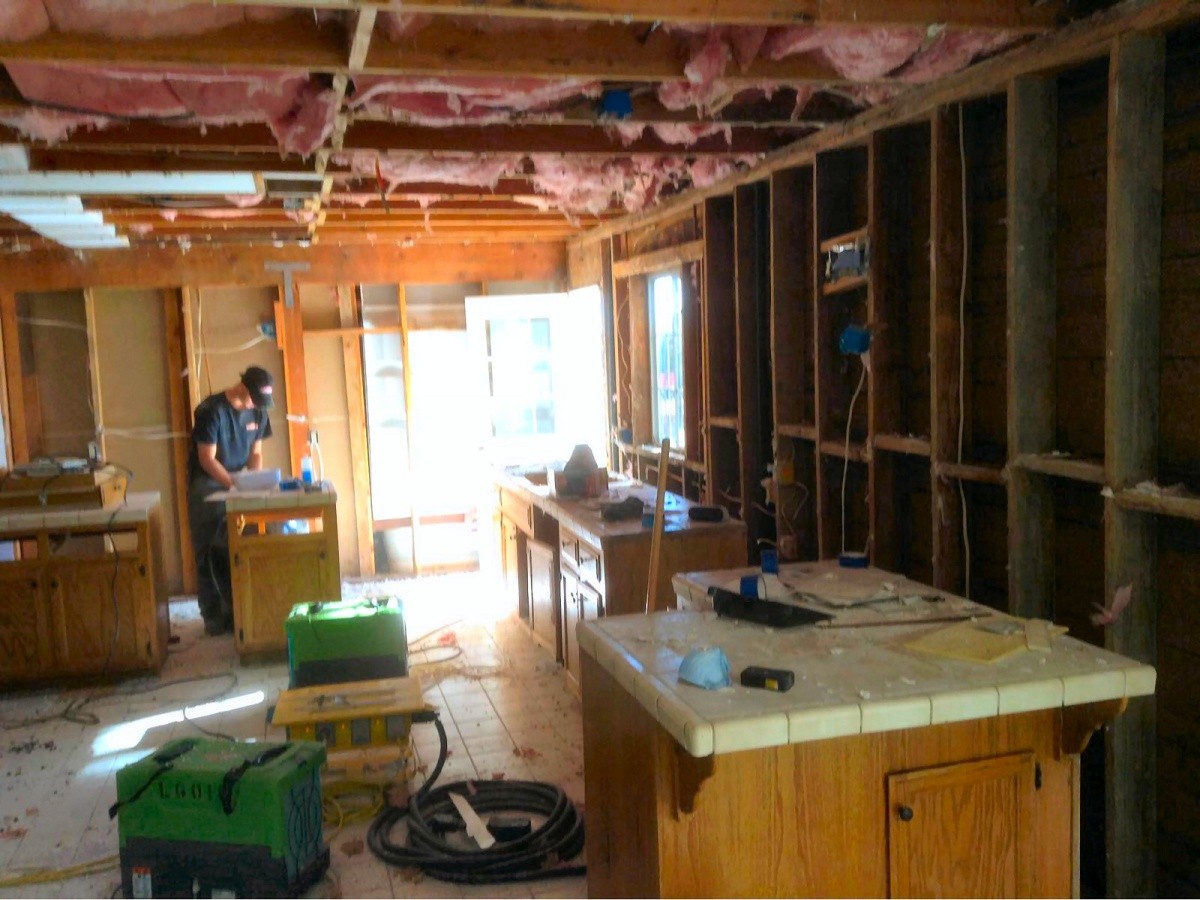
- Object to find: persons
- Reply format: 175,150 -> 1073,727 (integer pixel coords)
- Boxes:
187,366 -> 276,637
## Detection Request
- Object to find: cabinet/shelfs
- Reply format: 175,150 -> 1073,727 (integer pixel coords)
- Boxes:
225,482 -> 341,657
497,470 -> 747,701
577,645 -> 1080,899
1,492 -> 171,686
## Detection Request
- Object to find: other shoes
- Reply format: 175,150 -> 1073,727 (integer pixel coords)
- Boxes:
205,617 -> 232,635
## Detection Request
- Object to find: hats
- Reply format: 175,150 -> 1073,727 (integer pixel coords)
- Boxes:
242,365 -> 274,406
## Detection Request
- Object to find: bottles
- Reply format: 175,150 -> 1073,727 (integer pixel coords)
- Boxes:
310,432 -> 324,483
301,455 -> 313,486
